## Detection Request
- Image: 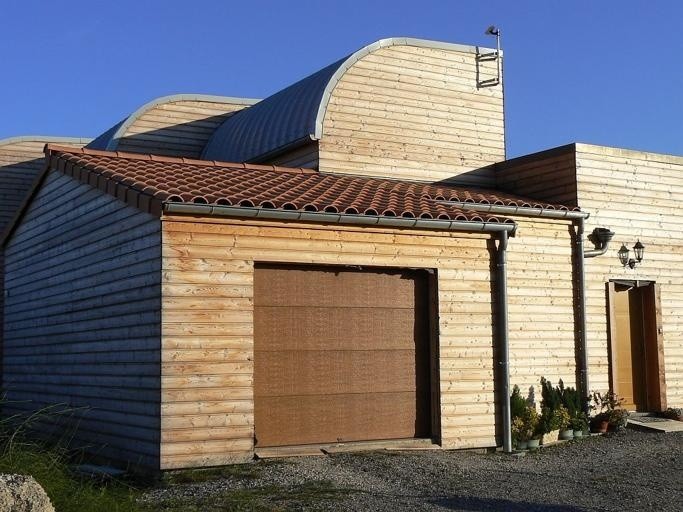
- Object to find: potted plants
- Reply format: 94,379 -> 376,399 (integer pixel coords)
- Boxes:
510,377 -> 629,449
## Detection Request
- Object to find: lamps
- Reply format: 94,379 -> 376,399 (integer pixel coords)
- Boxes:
617,242 -> 629,267
629,238 -> 645,268
